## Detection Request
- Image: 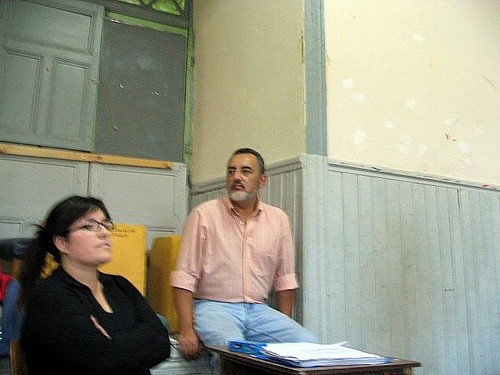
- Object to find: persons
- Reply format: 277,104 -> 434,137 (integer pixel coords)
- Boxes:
169,148 -> 318,375
14,196 -> 171,375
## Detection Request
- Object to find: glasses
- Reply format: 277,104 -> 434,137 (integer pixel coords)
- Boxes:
69,221 -> 113,231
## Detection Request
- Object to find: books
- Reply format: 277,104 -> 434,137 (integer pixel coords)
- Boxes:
228,335 -> 393,367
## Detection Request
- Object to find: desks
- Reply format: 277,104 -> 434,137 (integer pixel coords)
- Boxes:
206,345 -> 421,375
